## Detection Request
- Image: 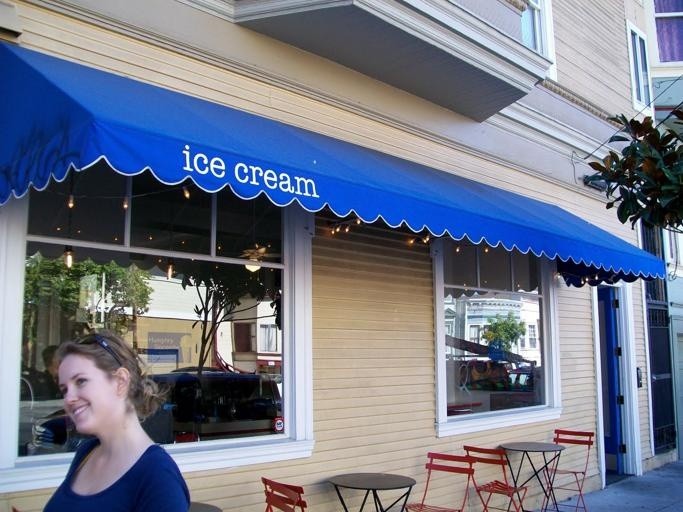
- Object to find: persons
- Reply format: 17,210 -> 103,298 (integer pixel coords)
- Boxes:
42,330 -> 192,511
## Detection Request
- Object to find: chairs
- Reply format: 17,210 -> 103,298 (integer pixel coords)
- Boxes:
261,476 -> 307,512
405,452 -> 477,512
463,445 -> 528,512
541,429 -> 594,512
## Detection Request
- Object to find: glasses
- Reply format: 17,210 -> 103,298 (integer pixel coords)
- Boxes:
74,333 -> 123,367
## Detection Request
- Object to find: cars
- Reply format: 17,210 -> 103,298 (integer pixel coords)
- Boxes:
456,360 -> 536,392
20,366 -> 283,455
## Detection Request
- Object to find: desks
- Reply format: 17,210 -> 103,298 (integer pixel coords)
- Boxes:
328,472 -> 416,512
187,502 -> 223,512
500,442 -> 565,512
448,402 -> 481,415
